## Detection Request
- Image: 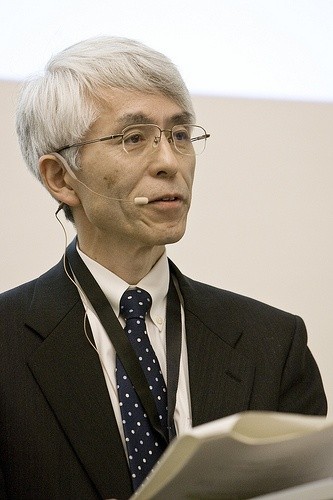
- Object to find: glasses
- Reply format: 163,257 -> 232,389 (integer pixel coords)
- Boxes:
55,124 -> 210,156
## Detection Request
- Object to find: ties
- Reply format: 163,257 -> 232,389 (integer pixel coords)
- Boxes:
116,290 -> 177,492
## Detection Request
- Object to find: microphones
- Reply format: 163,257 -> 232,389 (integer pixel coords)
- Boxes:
52,152 -> 148,204
